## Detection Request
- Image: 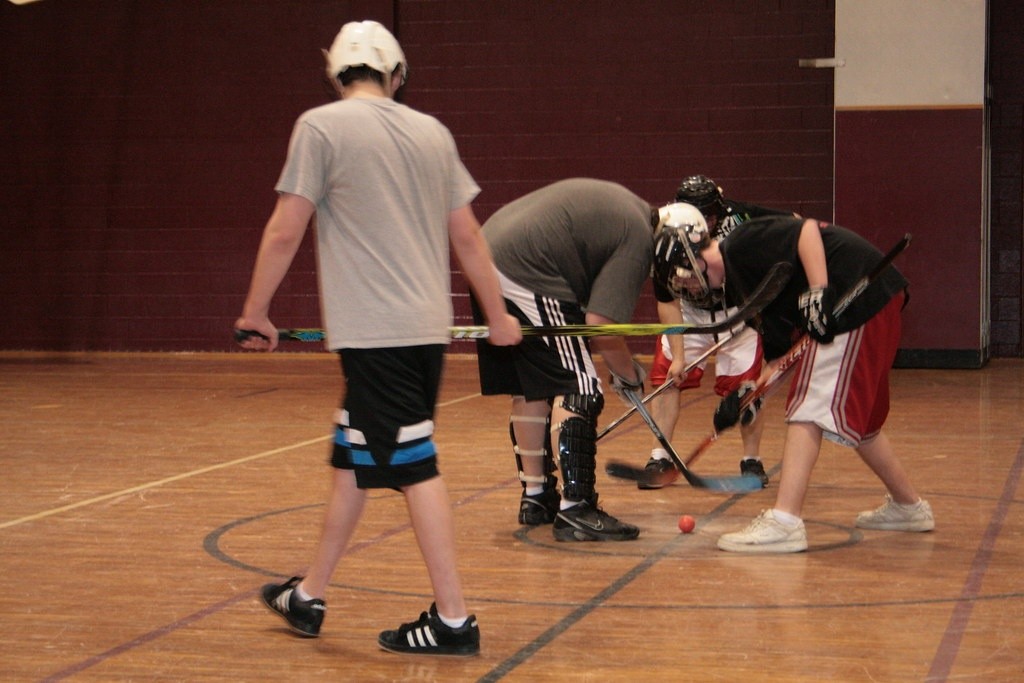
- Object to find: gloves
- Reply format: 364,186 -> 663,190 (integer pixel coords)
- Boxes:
799,290 -> 836,344
713,380 -> 764,433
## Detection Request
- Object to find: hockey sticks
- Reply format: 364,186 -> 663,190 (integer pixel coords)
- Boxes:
605,232 -> 912,480
597,323 -> 747,441
233,260 -> 794,343
622,388 -> 761,493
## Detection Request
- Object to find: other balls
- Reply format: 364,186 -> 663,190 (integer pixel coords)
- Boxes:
678,515 -> 695,533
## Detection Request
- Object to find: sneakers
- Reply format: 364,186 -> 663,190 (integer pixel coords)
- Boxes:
260,577 -> 324,637
639,457 -> 679,488
519,476 -> 565,524
716,508 -> 807,553
856,495 -> 936,532
741,458 -> 768,485
378,602 -> 480,656
551,492 -> 639,542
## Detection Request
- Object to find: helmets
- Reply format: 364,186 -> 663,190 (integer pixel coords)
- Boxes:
323,20 -> 408,88
675,174 -> 723,213
657,205 -> 709,233
651,234 -> 709,303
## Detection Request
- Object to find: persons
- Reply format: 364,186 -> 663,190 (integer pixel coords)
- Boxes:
467,178 -> 711,541
234,20 -> 524,659
637,174 -> 801,489
652,216 -> 935,553
